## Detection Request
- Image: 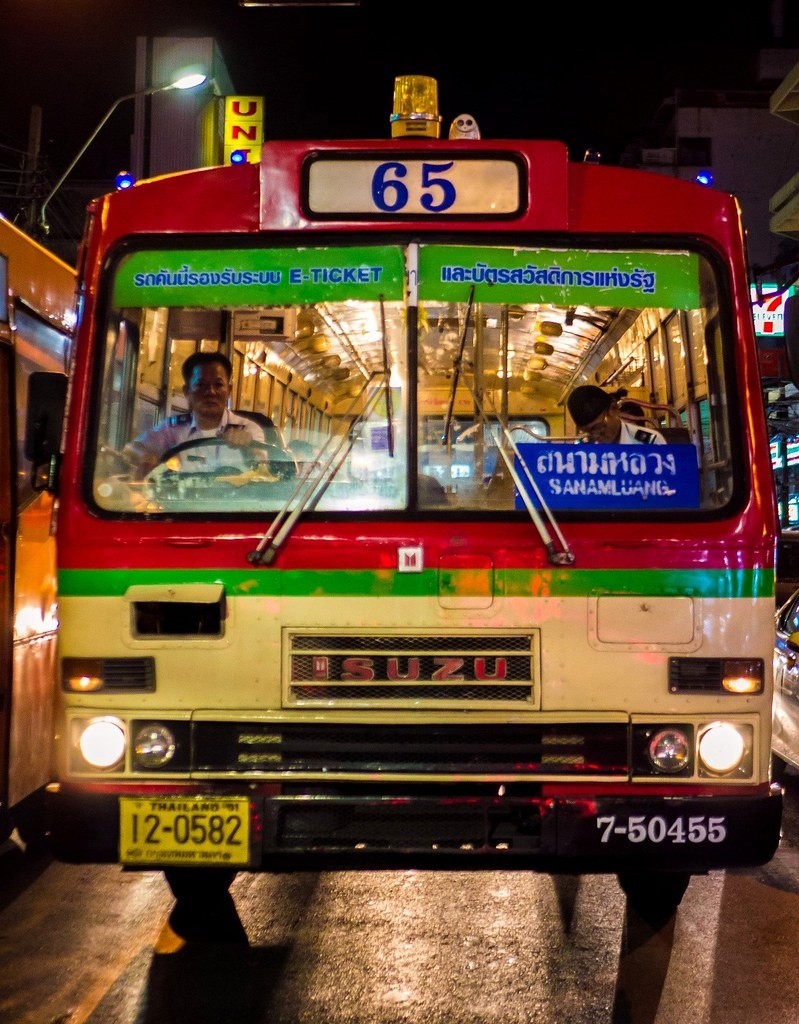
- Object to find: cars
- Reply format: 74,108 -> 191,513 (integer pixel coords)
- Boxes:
769,586 -> 799,783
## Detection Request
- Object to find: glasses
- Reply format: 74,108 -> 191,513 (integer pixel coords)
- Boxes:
584,416 -> 608,437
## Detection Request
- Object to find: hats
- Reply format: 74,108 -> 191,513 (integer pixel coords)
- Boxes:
567,385 -> 610,427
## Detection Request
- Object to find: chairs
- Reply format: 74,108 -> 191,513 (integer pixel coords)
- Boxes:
229,409 -> 282,480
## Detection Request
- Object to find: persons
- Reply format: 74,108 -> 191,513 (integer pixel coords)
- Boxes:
123,352 -> 268,476
287,440 -> 314,478
567,385 -> 668,444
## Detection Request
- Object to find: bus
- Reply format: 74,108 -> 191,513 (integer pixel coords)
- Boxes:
52,110 -> 783,920
0,213 -> 90,844
